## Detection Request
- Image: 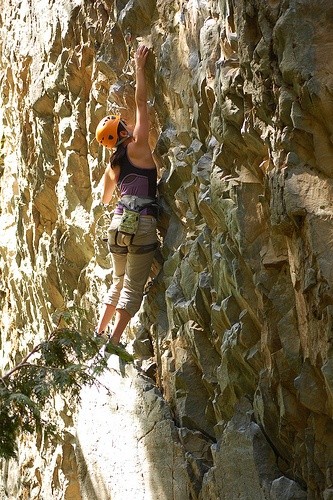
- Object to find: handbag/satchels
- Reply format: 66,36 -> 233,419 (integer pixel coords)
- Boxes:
118,208 -> 140,236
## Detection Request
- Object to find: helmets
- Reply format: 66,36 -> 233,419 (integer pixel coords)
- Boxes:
95,115 -> 121,149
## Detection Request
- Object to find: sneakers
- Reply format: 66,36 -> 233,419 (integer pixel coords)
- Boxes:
94,332 -> 108,344
105,342 -> 133,363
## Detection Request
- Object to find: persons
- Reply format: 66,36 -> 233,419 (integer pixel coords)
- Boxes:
93,45 -> 161,363
